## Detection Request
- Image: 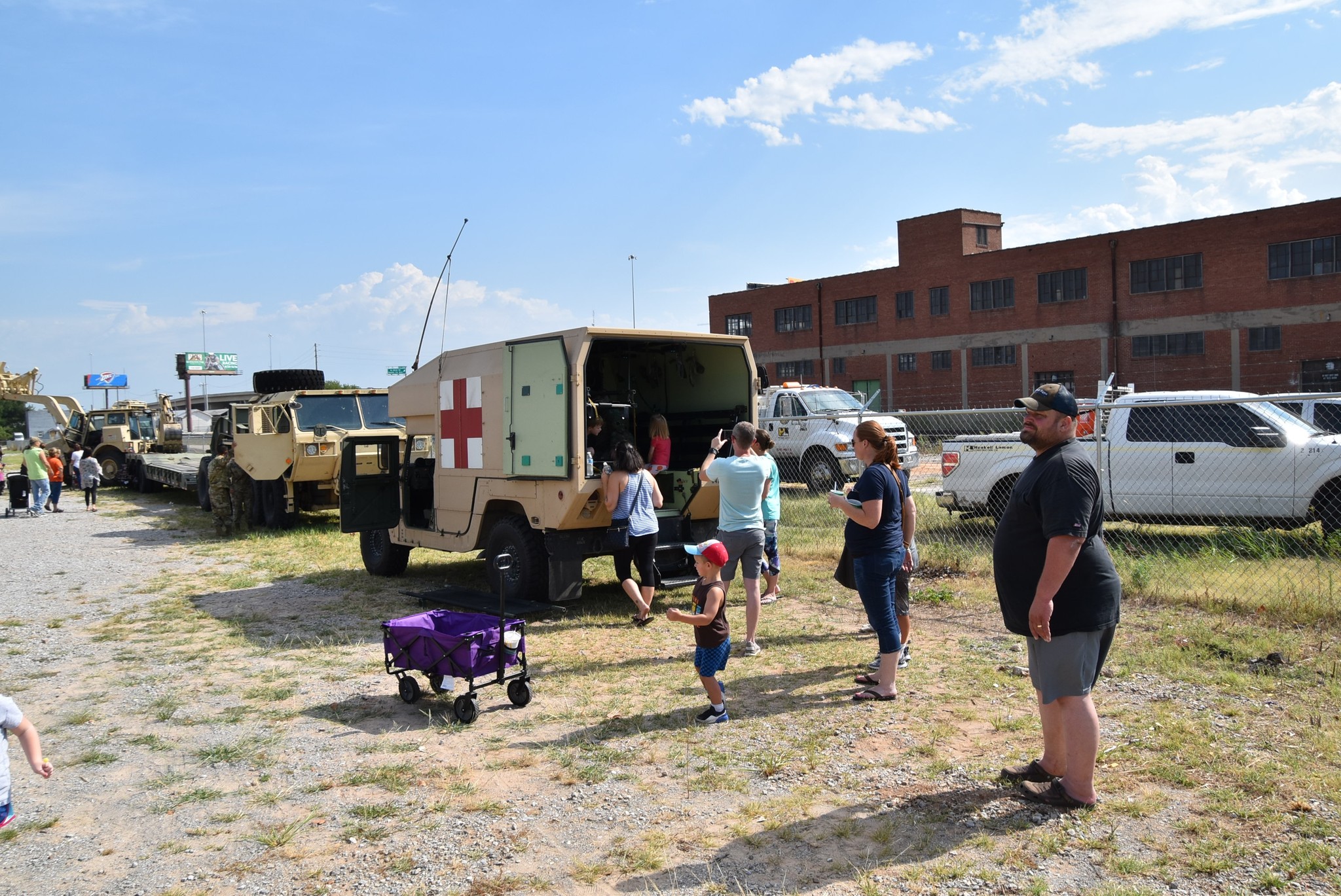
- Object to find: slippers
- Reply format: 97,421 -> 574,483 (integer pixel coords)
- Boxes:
632,615 -> 654,627
852,689 -> 896,701
760,592 -> 784,604
1001,758 -> 1065,783
854,673 -> 880,685
1018,777 -> 1095,809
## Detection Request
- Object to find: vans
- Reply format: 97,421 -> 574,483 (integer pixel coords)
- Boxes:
13,432 -> 25,441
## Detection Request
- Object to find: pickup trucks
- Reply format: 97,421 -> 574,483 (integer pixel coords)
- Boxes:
934,391 -> 1341,540
756,382 -> 919,496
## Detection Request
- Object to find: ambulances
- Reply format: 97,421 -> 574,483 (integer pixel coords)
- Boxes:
336,326 -> 760,615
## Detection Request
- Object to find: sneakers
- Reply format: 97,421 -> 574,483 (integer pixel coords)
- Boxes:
696,705 -> 728,723
869,645 -> 911,670
744,641 -> 760,656
707,681 -> 726,708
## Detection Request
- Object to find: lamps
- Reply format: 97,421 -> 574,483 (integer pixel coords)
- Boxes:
1048,335 -> 1054,340
862,349 -> 865,354
1327,313 -> 1331,320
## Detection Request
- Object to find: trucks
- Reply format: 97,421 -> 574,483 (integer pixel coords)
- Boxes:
125,369 -> 433,530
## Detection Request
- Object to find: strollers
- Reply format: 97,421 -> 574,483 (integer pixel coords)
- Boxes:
5,472 -> 32,518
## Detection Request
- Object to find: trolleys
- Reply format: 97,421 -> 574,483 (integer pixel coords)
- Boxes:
378,555 -> 532,724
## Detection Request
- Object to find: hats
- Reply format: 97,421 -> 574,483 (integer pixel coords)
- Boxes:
1014,383 -> 1078,417
684,539 -> 728,567
29,437 -> 43,443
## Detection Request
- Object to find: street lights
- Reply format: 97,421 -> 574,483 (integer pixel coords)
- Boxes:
89,352 -> 94,412
269,333 -> 272,372
199,309 -> 208,412
628,254 -> 637,329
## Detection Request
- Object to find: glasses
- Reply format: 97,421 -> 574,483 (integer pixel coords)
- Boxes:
851,439 -> 862,447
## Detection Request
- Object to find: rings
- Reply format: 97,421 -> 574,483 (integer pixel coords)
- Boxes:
1037,625 -> 1042,628
828,500 -> 830,503
908,562 -> 910,564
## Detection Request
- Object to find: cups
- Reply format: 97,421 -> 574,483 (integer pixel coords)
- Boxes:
847,499 -> 862,509
504,631 -> 521,651
829,489 -> 844,504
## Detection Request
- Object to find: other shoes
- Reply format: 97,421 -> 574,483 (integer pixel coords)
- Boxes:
53,508 -> 64,512
862,623 -> 878,638
44,505 -> 52,511
92,508 -> 97,511
29,509 -> 48,517
86,509 -> 92,511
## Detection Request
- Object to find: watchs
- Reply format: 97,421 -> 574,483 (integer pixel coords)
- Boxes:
709,448 -> 717,455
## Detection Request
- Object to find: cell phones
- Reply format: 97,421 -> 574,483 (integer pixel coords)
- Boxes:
720,430 -> 733,440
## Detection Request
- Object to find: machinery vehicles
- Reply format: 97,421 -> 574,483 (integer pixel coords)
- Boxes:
0,362 -> 188,488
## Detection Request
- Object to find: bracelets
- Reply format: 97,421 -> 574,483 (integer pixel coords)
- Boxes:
903,540 -> 910,549
712,447 -> 719,453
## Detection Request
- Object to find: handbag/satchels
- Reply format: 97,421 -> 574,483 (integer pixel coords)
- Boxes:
834,544 -> 858,592
607,518 -> 629,549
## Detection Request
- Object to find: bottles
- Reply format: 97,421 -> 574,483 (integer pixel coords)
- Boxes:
603,462 -> 613,476
587,452 -> 593,476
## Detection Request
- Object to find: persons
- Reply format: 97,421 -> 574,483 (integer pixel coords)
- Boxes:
207,442 -> 256,537
79,446 -> 104,512
0,693 -> 54,830
750,429 -> 781,604
205,352 -> 219,370
20,437 -> 54,518
647,414 -> 671,477
70,443 -> 84,492
993,385 -> 1120,810
699,421 -> 770,657
666,539 -> 731,724
43,448 -> 64,513
602,440 -> 664,626
587,413 -> 604,457
827,420 -> 920,701
0,445 -> 5,496
